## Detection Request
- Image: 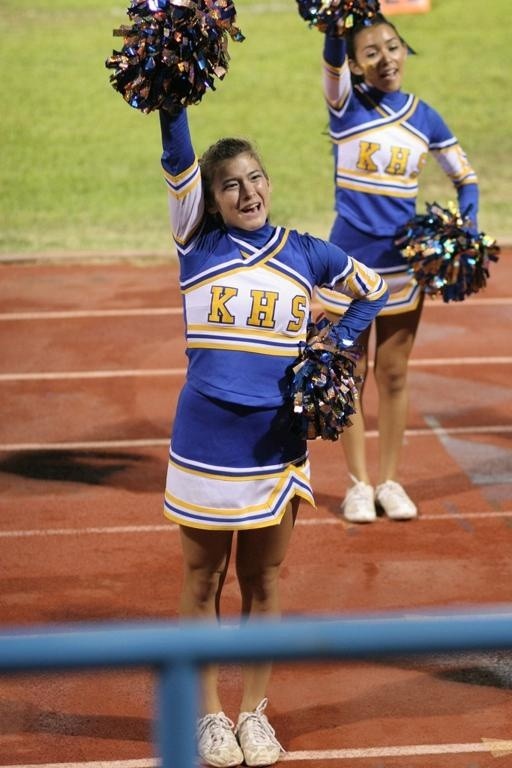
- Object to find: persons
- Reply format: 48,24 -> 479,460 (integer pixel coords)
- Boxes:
158,109 -> 389,767
315,13 -> 479,523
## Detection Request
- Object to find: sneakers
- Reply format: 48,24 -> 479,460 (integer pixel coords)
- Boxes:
343,481 -> 418,523
199,712 -> 280,767
